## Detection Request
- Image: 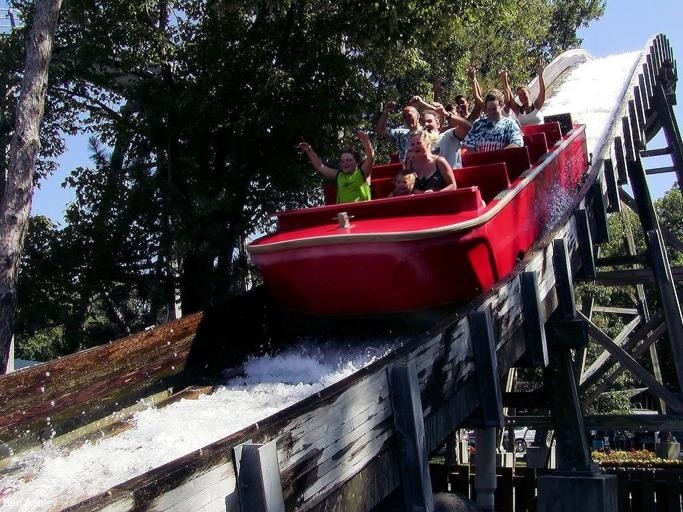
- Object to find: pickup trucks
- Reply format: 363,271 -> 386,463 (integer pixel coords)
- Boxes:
505,421 -> 540,449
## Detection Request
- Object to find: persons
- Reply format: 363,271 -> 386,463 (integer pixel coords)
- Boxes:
388,170 -> 433,197
390,129 -> 457,192
377,95 -> 438,162
464,92 -> 525,153
432,67 -> 482,131
298,132 -> 375,204
421,102 -> 472,169
469,68 -> 512,118
454,93 -> 471,119
499,58 -> 545,125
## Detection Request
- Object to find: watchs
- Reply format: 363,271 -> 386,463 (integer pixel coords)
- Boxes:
446,112 -> 452,120
